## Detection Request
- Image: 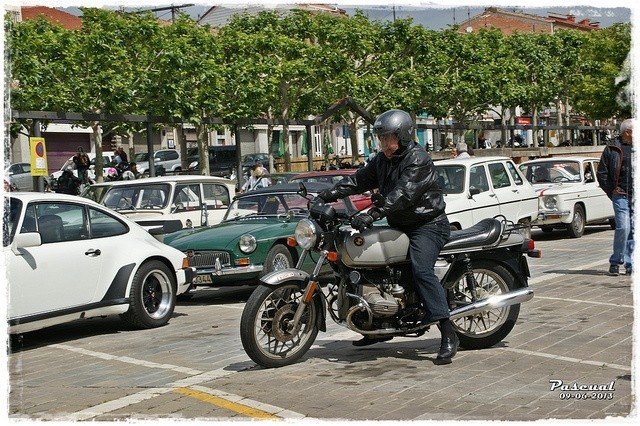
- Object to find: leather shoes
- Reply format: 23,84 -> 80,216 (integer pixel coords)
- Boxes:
352,335 -> 393,346
436,334 -> 460,361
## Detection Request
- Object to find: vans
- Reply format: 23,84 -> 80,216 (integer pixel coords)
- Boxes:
185,144 -> 240,176
430,156 -> 538,234
129,148 -> 183,175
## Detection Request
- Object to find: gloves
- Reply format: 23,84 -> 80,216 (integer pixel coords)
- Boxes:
317,188 -> 338,203
351,213 -> 374,234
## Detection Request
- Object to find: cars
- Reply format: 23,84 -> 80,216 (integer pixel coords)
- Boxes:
50,151 -> 123,190
5,162 -> 49,191
518,156 -> 623,238
5,192 -> 197,344
239,166 -> 297,208
164,183 -> 392,296
239,152 -> 278,174
43,180 -> 117,223
254,170 -> 379,214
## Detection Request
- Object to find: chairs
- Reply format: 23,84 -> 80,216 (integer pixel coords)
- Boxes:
470,173 -> 480,195
147,197 -> 162,206
534,168 -> 550,182
10,216 -> 36,243
263,198 -> 279,214
38,215 -> 65,244
453,171 -> 463,189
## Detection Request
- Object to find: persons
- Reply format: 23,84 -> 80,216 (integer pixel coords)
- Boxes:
236,162 -> 271,196
308,110 -> 460,365
108,146 -> 139,180
596,118 -> 632,276
73,147 -> 90,186
58,167 -> 81,195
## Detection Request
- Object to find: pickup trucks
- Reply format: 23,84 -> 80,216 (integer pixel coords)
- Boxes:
82,175 -> 266,233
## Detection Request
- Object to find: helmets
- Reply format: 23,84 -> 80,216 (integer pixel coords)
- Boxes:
373,109 -> 414,147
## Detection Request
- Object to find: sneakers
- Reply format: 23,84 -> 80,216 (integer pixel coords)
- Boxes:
624,263 -> 632,276
608,265 -> 619,276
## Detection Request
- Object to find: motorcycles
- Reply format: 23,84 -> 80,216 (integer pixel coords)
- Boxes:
240,183 -> 541,368
